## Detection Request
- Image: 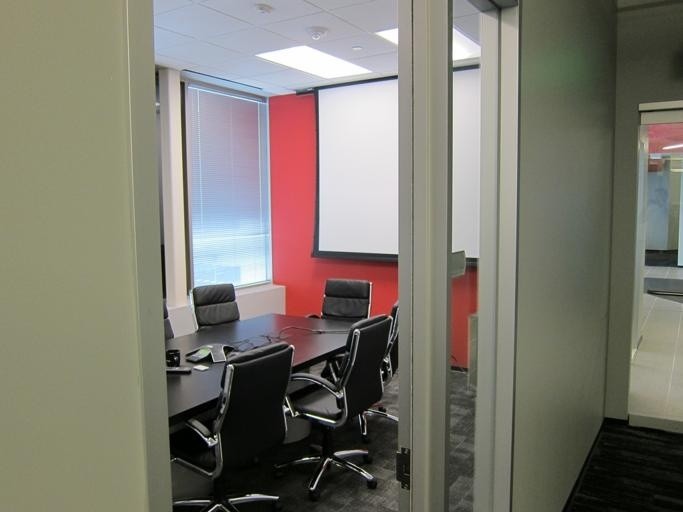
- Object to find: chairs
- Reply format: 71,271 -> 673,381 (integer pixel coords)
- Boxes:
334,300 -> 400,444
306,279 -> 372,322
286,314 -> 392,501
190,283 -> 240,332
170,341 -> 296,512
163,299 -> 174,340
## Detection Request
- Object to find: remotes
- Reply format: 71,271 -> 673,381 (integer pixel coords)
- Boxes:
166,366 -> 193,373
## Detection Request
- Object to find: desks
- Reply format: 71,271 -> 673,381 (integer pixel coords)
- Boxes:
165,313 -> 354,475
